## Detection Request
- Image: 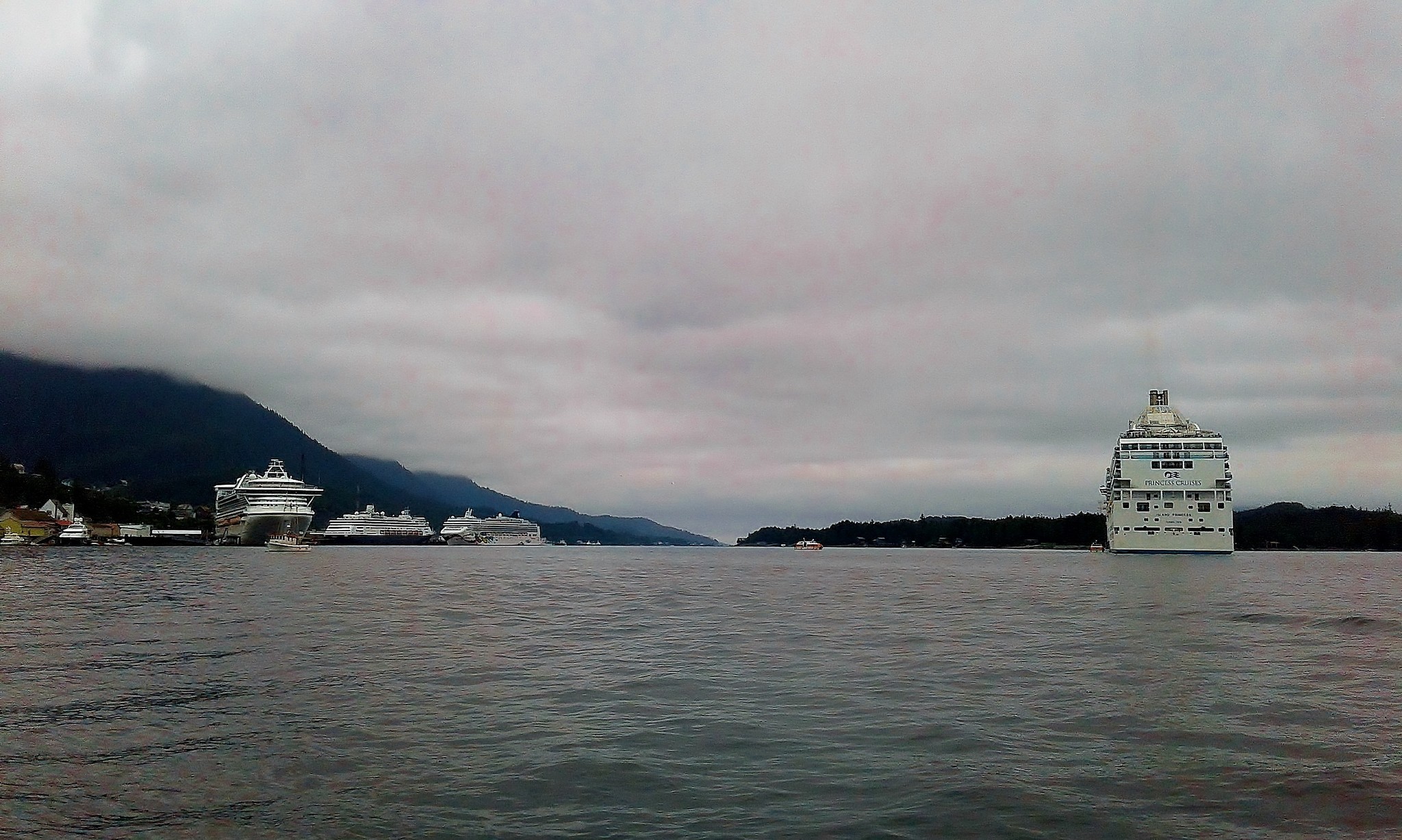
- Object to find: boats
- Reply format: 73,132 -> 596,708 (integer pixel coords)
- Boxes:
553,538 -> 568,546
383,531 -> 423,535
28,541 -> 39,546
264,529 -> 313,552
1090,540 -> 1105,554
90,538 -> 133,546
795,539 -> 825,551
481,532 -> 527,537
123,532 -> 209,547
58,517 -> 91,545
0,526 -> 26,546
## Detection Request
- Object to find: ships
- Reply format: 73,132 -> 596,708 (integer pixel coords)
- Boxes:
321,504 -> 437,546
214,458 -> 325,546
440,507 -> 542,546
1097,388 -> 1237,555
